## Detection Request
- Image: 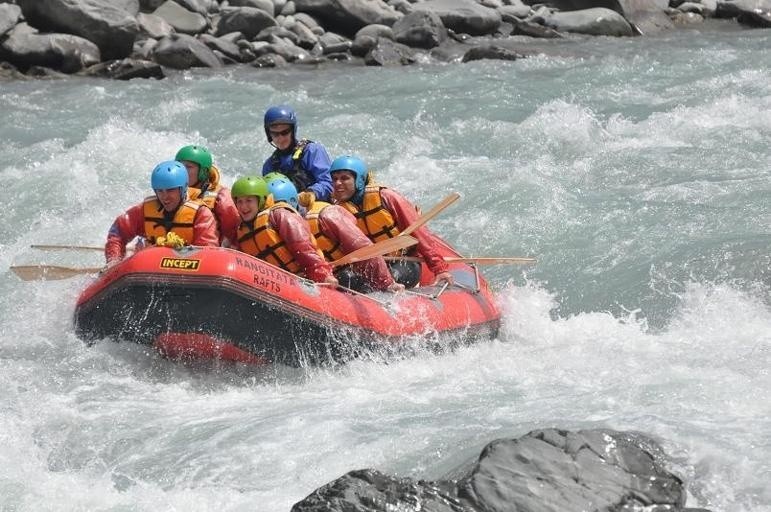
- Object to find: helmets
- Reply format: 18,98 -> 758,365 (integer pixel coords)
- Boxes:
232,172 -> 299,210
175,146 -> 212,180
152,161 -> 188,201
264,105 -> 296,142
330,155 -> 368,193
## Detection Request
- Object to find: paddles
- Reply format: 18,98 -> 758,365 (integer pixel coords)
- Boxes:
330,193 -> 460,267
11,265 -> 105,281
384,256 -> 534,265
31,244 -> 133,252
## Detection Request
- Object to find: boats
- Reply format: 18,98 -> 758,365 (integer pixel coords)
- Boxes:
72,229 -> 504,382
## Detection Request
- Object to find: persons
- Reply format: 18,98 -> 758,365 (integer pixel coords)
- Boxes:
226,176 -> 340,288
104,159 -> 220,275
175,144 -> 238,249
330,154 -> 455,291
265,174 -> 405,296
262,105 -> 334,201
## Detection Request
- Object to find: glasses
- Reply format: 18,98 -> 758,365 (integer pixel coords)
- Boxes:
268,129 -> 293,137
